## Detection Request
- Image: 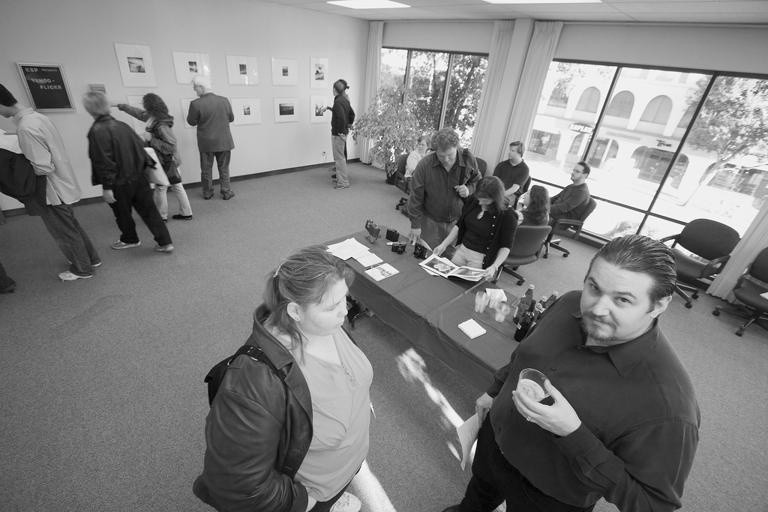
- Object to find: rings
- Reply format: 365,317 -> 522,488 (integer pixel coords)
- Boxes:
525,415 -> 532,423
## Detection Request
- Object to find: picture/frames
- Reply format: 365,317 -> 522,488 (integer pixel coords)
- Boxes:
274,98 -> 299,123
181,98 -> 199,129
173,51 -> 211,87
226,54 -> 260,85
125,94 -> 152,136
16,63 -> 77,114
230,99 -> 262,126
310,57 -> 330,89
113,43 -> 157,89
271,58 -> 297,86
311,96 -> 334,124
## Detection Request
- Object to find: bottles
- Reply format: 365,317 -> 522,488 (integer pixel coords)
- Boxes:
514,283 -> 535,325
514,300 -> 536,342
547,290 -> 558,308
535,296 -> 547,309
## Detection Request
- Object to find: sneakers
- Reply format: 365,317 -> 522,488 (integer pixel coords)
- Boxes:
90,261 -> 103,269
331,490 -> 362,512
153,243 -> 175,253
58,270 -> 93,281
112,240 -> 142,250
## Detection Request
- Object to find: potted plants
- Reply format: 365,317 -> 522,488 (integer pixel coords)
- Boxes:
352,85 -> 427,184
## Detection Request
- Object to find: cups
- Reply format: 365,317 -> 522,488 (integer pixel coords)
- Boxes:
474,291 -> 489,313
517,369 -> 553,402
494,303 -> 511,321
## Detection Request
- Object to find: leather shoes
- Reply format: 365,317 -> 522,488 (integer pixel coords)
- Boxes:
172,214 -> 193,221
205,193 -> 214,200
223,191 -> 235,201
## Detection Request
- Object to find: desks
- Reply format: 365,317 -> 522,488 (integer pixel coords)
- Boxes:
322,227 -> 541,395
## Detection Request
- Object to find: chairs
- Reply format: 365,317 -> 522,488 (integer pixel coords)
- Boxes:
657,218 -> 741,309
712,246 -> 768,337
396,154 -> 413,210
474,155 -> 488,179
537,195 -> 597,260
491,226 -> 551,286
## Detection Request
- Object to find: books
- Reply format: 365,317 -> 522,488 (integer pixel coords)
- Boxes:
418,253 -> 488,283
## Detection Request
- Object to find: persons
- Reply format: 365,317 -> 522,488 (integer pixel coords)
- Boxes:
0,84 -> 103,282
405,128 -> 482,250
109,94 -> 194,221
404,135 -> 431,192
493,141 -> 530,211
434,175 -> 518,280
548,161 -> 590,229
192,248 -> 397,512
440,234 -> 701,512
186,75 -> 235,201
321,79 -> 362,189
82,90 -> 174,253
515,184 -> 548,227
